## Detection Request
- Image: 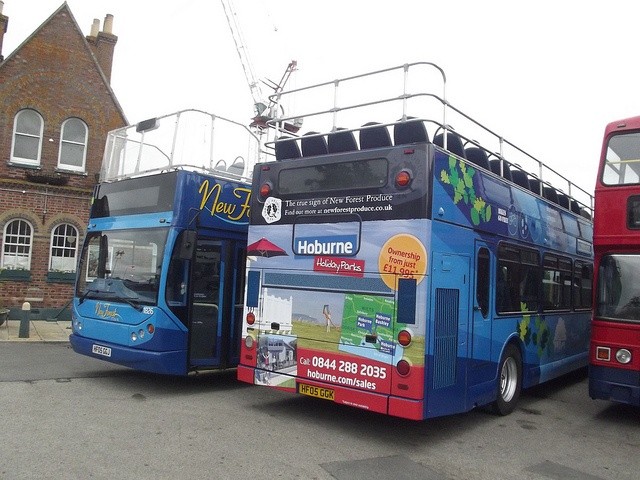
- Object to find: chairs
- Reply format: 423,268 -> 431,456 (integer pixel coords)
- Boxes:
394,116 -> 429,146
542,181 -> 562,205
328,127 -> 359,153
433,125 -> 468,160
527,172 -> 548,199
508,163 -> 531,190
487,153 -> 514,182
556,190 -> 571,210
570,198 -> 581,215
301,131 -> 328,157
275,136 -> 302,160
210,160 -> 227,178
463,140 -> 492,171
226,156 -> 246,181
360,122 -> 392,149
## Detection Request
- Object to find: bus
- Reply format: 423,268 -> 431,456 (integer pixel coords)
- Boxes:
235,61 -> 596,421
586,115 -> 640,408
69,108 -> 259,385
